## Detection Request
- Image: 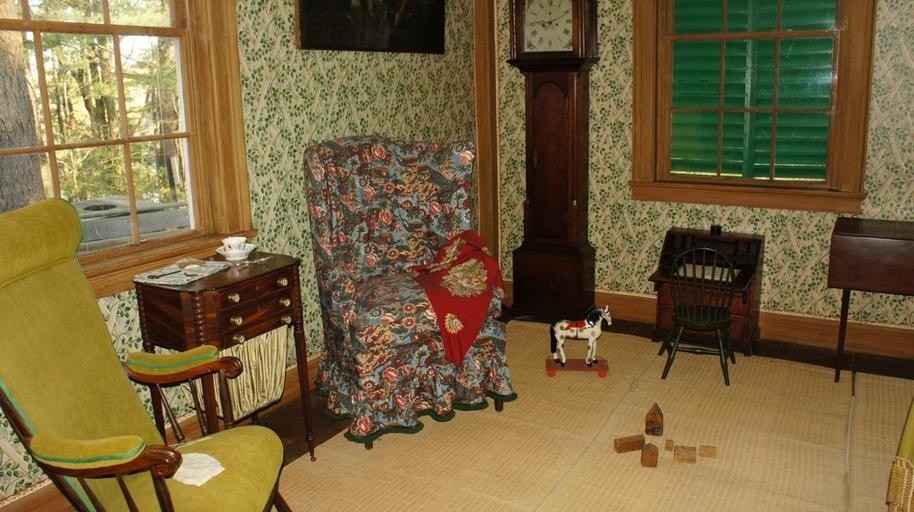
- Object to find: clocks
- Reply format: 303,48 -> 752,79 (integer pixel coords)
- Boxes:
508,0 -> 602,319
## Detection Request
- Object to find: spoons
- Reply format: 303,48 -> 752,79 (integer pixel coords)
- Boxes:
147,270 -> 182,281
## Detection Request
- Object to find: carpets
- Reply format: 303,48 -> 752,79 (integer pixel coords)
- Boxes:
270,317 -> 912,512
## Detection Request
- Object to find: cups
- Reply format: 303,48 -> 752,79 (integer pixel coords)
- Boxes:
222,235 -> 246,251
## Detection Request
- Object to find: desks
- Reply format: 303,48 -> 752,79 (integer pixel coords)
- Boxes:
132,252 -> 316,465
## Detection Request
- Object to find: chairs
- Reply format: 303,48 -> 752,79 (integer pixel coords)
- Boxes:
658,247 -> 737,382
0,196 -> 294,512
301,136 -> 522,450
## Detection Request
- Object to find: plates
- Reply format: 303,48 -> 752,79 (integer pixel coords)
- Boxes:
215,244 -> 256,260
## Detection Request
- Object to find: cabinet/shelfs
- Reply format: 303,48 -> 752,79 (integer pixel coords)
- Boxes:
827,217 -> 914,382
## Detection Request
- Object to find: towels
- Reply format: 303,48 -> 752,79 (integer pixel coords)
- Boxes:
132,257 -> 232,287
